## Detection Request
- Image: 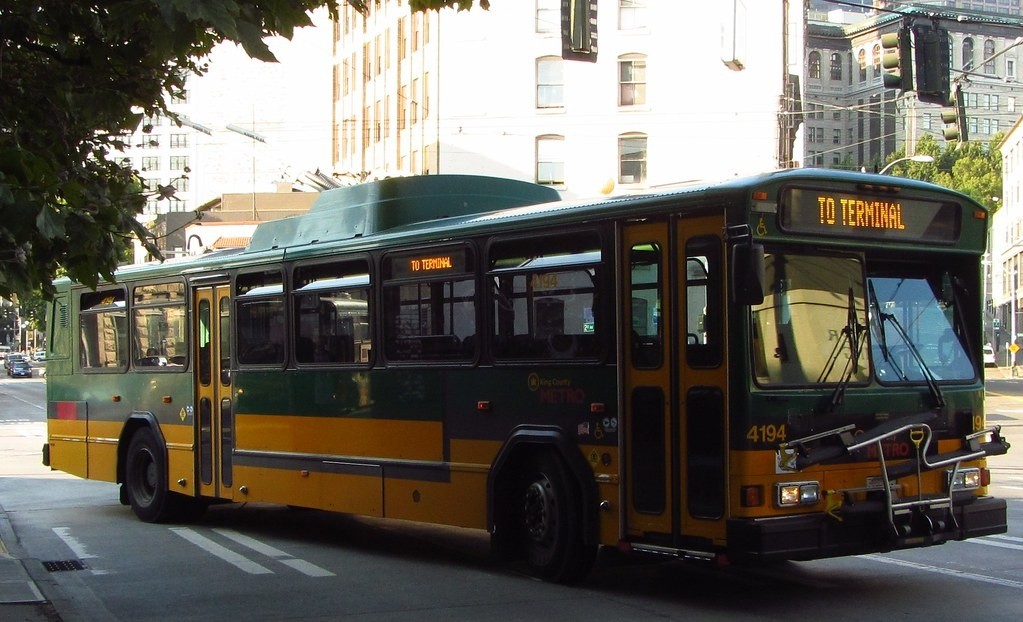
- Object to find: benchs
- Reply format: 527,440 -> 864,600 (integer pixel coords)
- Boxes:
545,329 -> 639,359
462,333 -> 539,360
631,333 -> 700,354
276,335 -> 355,364
396,335 -> 460,358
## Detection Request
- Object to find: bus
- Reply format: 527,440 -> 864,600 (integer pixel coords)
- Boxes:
41,171 -> 1011,589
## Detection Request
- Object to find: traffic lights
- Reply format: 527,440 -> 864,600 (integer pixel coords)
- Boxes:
940,84 -> 968,143
881,26 -> 913,92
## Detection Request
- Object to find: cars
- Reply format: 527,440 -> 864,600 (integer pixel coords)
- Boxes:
983,345 -> 996,366
0,345 -> 47,379
